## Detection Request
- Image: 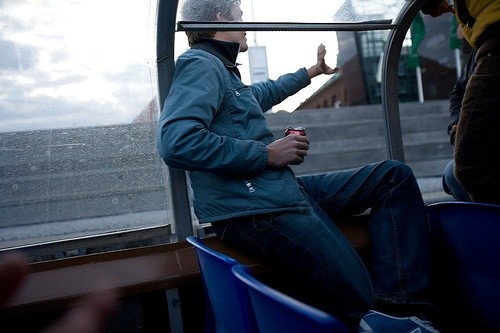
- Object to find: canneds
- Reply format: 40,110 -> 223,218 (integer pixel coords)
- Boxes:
284,126 -> 306,164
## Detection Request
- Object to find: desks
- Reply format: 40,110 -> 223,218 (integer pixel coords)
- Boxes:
0,208 -> 371,333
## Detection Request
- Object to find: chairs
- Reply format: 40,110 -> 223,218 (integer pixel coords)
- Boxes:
186,236 -> 254,333
230,266 -> 345,333
419,201 -> 500,329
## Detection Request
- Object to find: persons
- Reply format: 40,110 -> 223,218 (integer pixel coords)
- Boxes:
420,0 -> 500,204
155,0 -> 444,333
